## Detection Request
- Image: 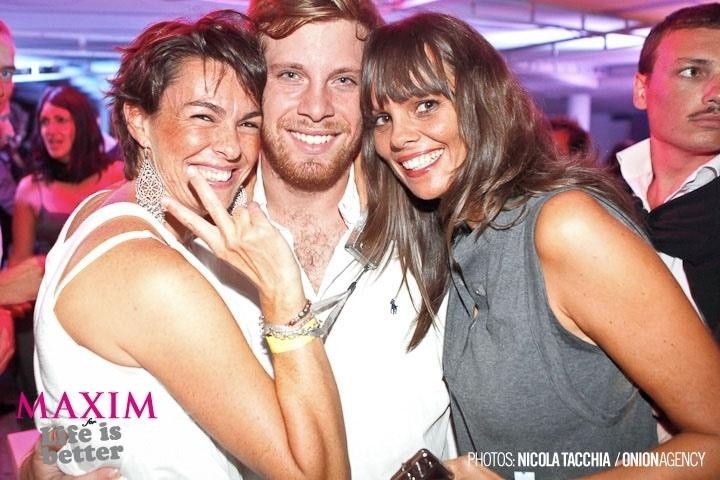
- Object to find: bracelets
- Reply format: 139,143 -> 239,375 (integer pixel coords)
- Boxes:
257,297 -> 324,356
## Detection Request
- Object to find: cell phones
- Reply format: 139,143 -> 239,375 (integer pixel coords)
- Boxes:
391,448 -> 455,480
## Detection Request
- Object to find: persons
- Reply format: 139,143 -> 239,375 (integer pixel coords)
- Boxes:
31,6 -> 352,480
0,19 -> 131,435
357,10 -> 720,480
542,114 -> 596,169
14,0 -> 459,480
599,2 -> 719,343
